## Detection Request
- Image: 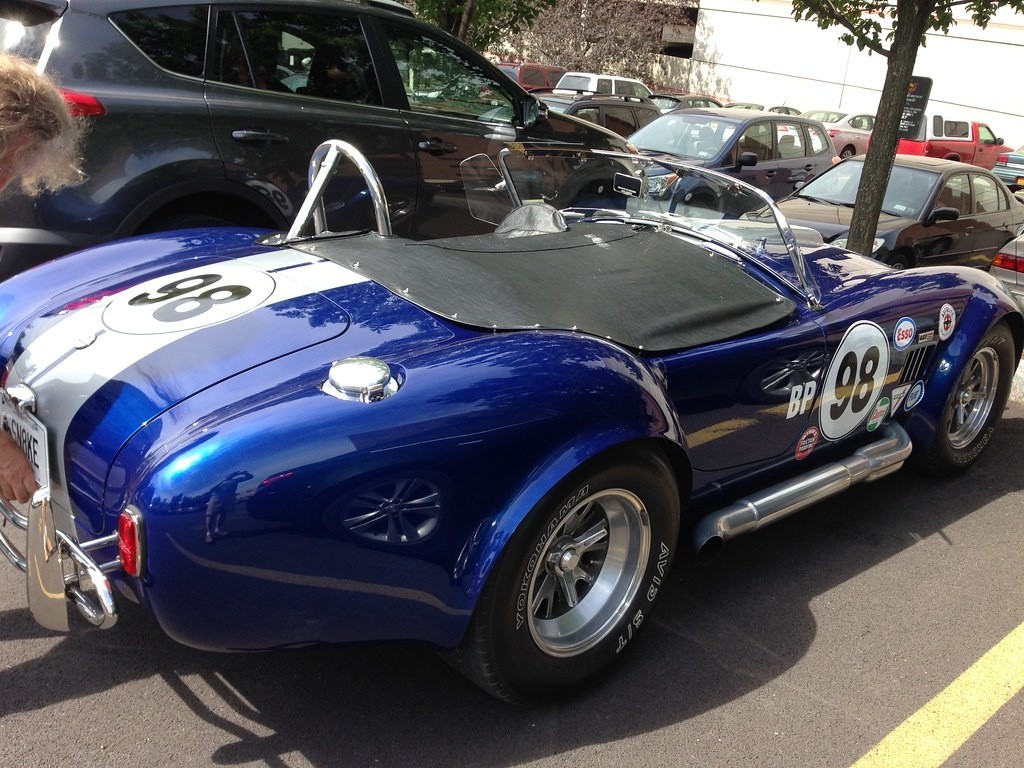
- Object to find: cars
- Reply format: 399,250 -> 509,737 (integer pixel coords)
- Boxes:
989,234 -> 1024,313
647,94 -> 722,114
990,145 -> 1024,185
739,151 -> 1024,277
708,101 -> 802,140
787,110 -> 878,160
0,135 -> 1024,711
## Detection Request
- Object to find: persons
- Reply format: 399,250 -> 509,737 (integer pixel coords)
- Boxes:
0,55 -> 94,504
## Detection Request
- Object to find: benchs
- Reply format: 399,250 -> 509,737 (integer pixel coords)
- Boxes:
778,135 -> 802,159
896,177 -> 962,210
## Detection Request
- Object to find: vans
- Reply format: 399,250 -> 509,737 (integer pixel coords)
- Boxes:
497,60 -> 569,91
626,108 -> 838,221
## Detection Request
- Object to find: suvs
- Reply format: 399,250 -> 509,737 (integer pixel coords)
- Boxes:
897,113 -> 1015,171
552,72 -> 658,104
481,86 -> 662,141
0,0 -> 637,269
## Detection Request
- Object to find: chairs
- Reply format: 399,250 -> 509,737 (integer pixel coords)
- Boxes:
696,126 -> 714,152
745,133 -> 766,162
309,44 -> 379,105
939,187 -> 952,207
250,32 -> 279,92
